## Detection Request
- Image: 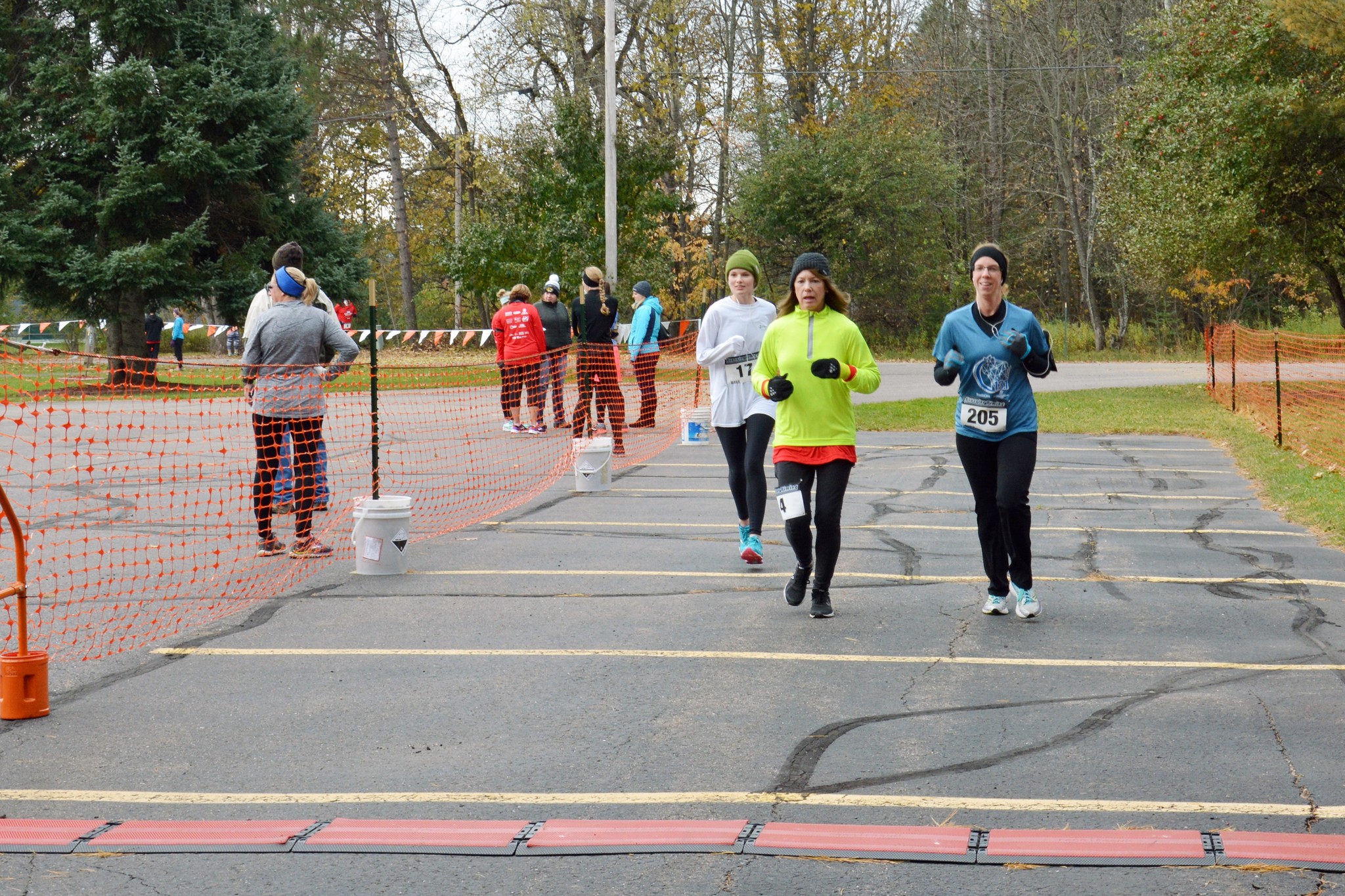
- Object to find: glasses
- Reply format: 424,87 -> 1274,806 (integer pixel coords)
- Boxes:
264,284 -> 278,291
972,266 -> 1003,274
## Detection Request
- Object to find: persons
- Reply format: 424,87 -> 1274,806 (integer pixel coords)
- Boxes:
226,326 -> 241,356
629,280 -> 664,428
752,252 -> 881,619
171,308 -> 185,371
334,299 -> 357,330
145,308 -> 164,370
572,266 -> 626,454
930,243 -> 1057,619
240,265 -> 358,558
242,240 -> 343,514
697,249 -> 777,564
491,284 -> 547,434
532,274 -> 571,430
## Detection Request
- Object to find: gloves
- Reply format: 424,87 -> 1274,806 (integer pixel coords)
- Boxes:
811,358 -> 841,379
943,344 -> 964,373
768,374 -> 794,403
999,328 -> 1031,360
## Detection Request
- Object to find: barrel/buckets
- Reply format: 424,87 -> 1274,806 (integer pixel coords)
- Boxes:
351,495 -> 412,574
570,437 -> 613,492
680,406 -> 712,445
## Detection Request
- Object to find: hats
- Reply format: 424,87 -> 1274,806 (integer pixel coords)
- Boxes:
544,274 -> 560,295
633,281 -> 651,298
790,252 -> 830,290
725,250 -> 761,289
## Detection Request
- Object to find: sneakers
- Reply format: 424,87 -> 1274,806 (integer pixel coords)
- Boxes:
739,523 -> 750,556
314,502 -> 328,511
502,418 -> 657,458
982,592 -> 1011,615
258,531 -> 287,556
271,503 -> 296,514
290,536 -> 334,559
1009,580 -> 1041,618
741,536 -> 763,564
784,561 -> 814,606
809,588 -> 834,619
